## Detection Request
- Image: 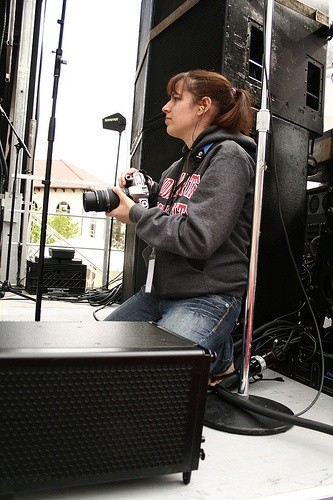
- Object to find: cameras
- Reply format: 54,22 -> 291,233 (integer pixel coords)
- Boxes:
83,172 -> 149,211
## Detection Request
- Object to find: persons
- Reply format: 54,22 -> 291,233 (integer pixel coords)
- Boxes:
97,68 -> 259,392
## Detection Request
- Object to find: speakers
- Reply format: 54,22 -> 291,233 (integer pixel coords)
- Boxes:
0,321 -> 217,496
